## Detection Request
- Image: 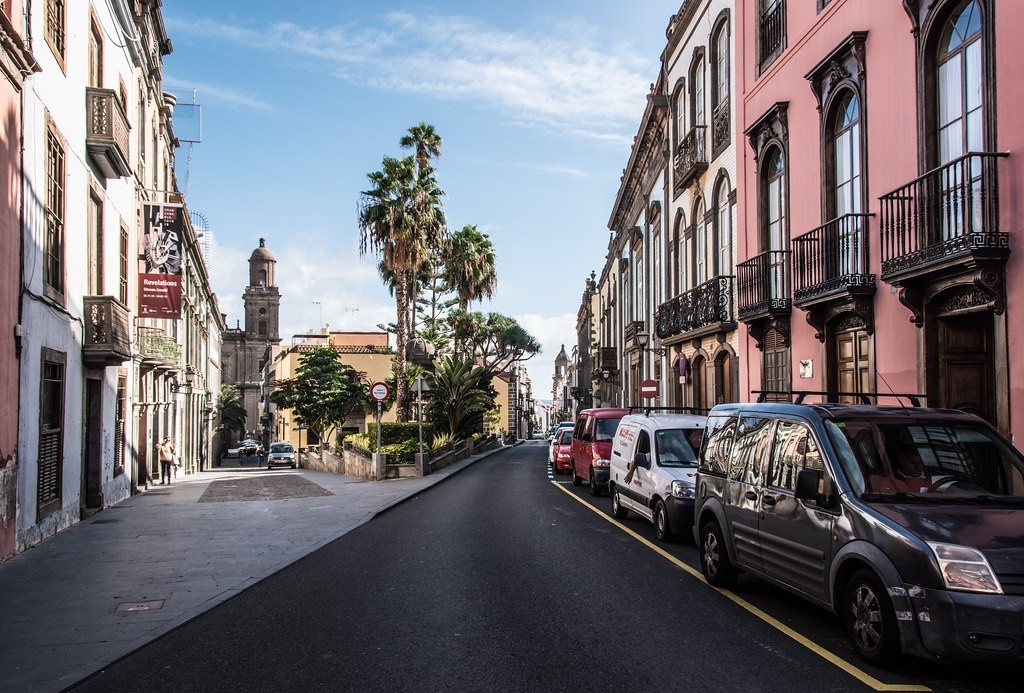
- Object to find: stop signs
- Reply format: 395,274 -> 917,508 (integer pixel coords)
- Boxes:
639,379 -> 659,400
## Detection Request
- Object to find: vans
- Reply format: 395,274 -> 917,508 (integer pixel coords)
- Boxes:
569,406 -> 638,497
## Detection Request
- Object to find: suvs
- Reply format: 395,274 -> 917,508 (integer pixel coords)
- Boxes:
692,389 -> 1023,676
608,405 -> 713,544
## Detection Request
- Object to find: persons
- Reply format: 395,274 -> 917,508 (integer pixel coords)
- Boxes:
877,449 -> 935,493
155,436 -> 176,485
254,443 -> 263,467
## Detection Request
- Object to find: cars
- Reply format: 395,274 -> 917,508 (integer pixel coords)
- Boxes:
531,430 -> 544,440
243,444 -> 259,455
547,421 -> 575,476
265,442 -> 297,470
